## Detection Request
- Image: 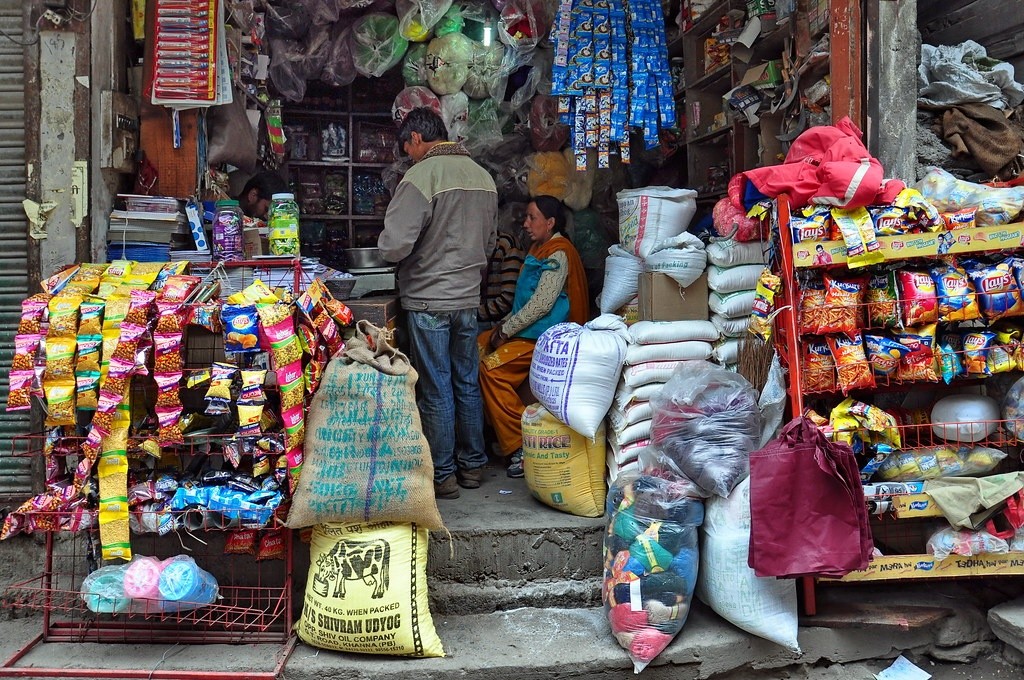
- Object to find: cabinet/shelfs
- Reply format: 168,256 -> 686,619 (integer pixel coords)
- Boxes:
766,197 -> 1023,616
661,0 -> 791,232
261,78 -> 397,249
0,259 -> 301,680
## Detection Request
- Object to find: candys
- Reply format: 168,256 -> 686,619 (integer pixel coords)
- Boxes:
269,207 -> 299,256
213,213 -> 243,261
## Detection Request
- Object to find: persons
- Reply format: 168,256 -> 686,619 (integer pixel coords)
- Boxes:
937,234 -> 947,254
477,230 -> 524,330
813,244 -> 832,265
377,106 -> 498,498
231,173 -> 289,222
477,195 -> 588,477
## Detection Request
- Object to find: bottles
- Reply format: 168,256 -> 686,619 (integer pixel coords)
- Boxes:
933,395 -> 997,442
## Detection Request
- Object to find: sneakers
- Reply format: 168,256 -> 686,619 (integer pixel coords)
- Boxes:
459,468 -> 483,489
433,474 -> 460,499
507,447 -> 525,477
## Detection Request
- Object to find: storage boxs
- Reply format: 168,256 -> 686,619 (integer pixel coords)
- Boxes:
638,272 -> 709,321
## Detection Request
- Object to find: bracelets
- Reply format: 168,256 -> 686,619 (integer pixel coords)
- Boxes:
499,328 -> 505,342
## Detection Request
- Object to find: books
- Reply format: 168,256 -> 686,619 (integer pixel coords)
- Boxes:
106,194 -> 323,301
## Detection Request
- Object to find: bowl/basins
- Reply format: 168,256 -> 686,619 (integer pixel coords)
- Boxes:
344,246 -> 387,266
